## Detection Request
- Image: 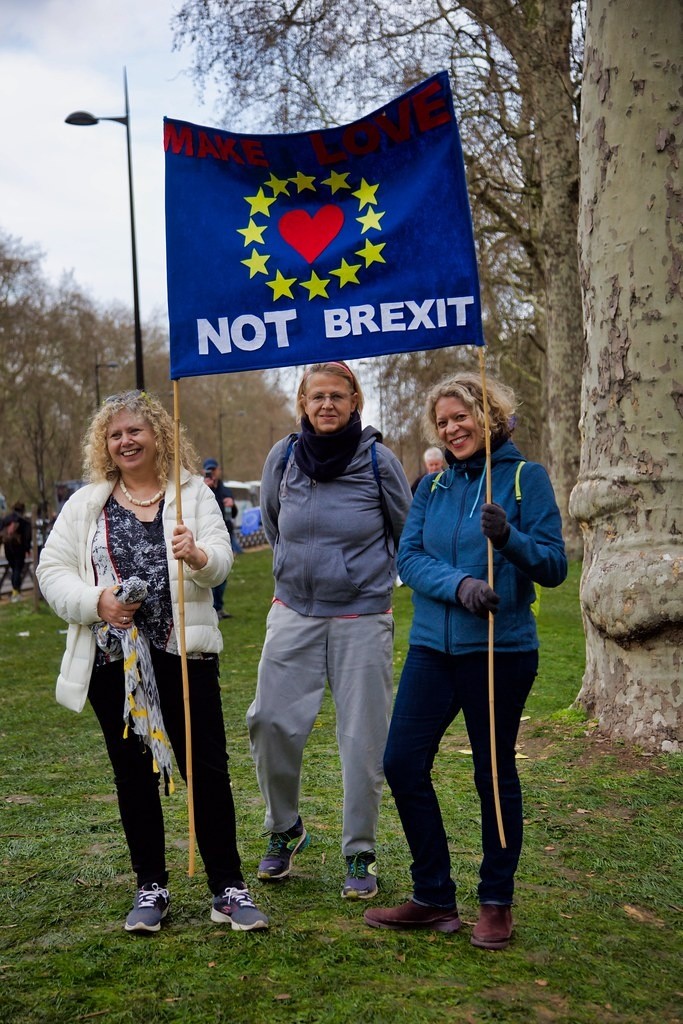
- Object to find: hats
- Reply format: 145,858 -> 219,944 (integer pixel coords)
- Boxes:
203,457 -> 219,470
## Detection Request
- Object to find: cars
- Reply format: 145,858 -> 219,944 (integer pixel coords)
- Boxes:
223,480 -> 261,530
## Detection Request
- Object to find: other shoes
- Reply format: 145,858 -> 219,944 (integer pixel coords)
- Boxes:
217,610 -> 233,620
11,594 -> 25,603
395,575 -> 406,588
363,899 -> 462,932
470,905 -> 514,949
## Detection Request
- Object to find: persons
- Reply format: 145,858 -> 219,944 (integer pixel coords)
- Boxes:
0,494 -> 54,602
410,447 -> 447,499
246,360 -> 413,900
202,457 -> 238,620
365,373 -> 568,949
35,390 -> 268,933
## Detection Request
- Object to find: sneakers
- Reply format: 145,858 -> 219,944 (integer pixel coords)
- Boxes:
257,814 -> 311,880
124,883 -> 170,932
209,880 -> 270,931
341,848 -> 379,899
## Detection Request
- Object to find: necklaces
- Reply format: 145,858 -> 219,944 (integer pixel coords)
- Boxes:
119,475 -> 166,507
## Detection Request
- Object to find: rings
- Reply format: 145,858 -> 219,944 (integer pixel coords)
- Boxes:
123,617 -> 128,624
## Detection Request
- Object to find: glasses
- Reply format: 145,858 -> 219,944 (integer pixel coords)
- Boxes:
301,393 -> 353,404
102,388 -> 155,410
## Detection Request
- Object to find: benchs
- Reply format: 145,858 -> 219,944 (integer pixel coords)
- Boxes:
0,558 -> 34,590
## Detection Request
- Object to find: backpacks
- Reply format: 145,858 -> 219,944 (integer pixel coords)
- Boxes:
0,518 -> 24,545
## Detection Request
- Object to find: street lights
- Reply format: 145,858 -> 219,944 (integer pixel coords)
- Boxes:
95,362 -> 119,409
65,111 -> 146,392
218,411 -> 245,480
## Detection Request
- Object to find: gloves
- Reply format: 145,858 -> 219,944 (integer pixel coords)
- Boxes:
456,576 -> 500,619
480,502 -> 510,550
